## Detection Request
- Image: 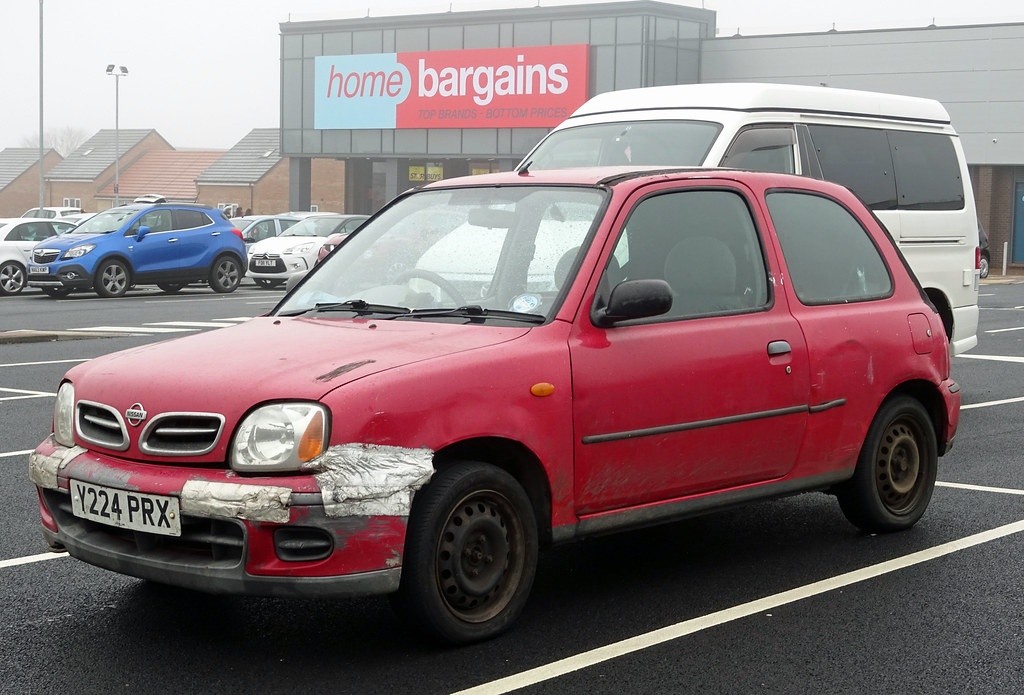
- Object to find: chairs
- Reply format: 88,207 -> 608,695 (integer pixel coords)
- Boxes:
657,236 -> 748,315
554,244 -> 620,294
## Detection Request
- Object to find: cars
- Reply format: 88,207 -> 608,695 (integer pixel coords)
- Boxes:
244,215 -> 371,290
0,216 -> 78,296
224,215 -> 316,255
52,212 -> 97,235
26,165 -> 960,640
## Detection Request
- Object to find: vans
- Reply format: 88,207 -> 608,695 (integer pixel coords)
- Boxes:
410,83 -> 980,359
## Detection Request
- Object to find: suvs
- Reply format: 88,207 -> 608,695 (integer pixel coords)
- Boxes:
26,193 -> 248,299
20,207 -> 85,220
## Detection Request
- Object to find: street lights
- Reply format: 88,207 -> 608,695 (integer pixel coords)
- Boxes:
107,65 -> 129,206
